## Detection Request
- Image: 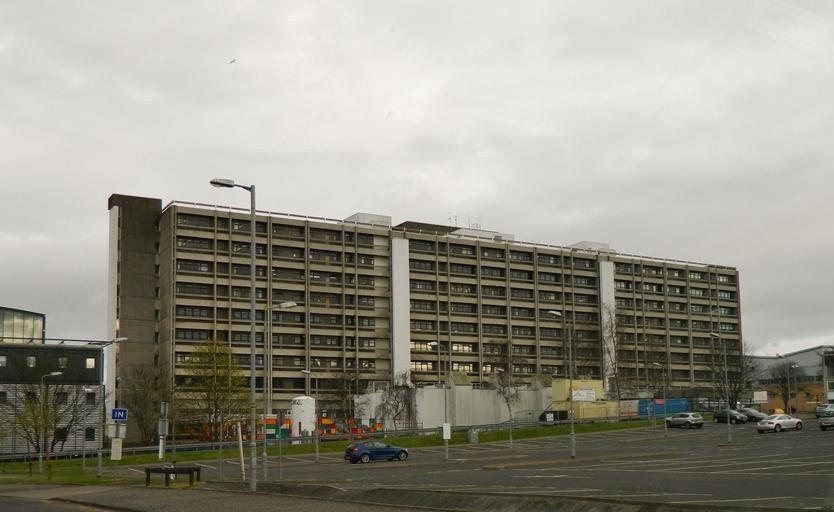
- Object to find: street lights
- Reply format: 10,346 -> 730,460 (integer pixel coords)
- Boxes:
209,176 -> 259,494
497,368 -> 514,450
263,301 -> 299,482
80,386 -> 93,469
776,353 -> 792,417
709,332 -> 732,443
789,360 -> 800,413
428,341 -> 451,462
545,310 -> 577,460
97,337 -> 129,479
37,371 -> 63,474
300,369 -> 321,465
652,362 -> 668,438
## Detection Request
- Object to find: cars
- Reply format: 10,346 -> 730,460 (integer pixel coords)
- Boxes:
735,407 -> 769,424
755,413 -> 805,435
817,409 -> 834,431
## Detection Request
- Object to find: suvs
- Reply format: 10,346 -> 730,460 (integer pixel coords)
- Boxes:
815,404 -> 834,420
342,439 -> 410,467
663,412 -> 705,431
713,408 -> 748,426
